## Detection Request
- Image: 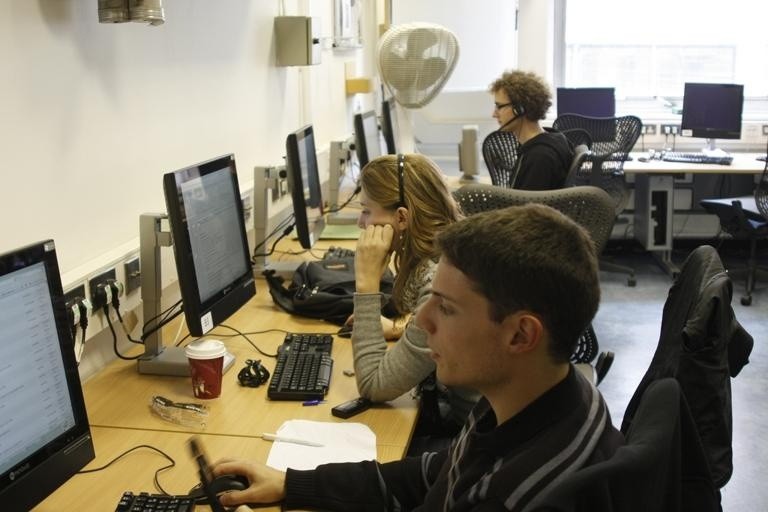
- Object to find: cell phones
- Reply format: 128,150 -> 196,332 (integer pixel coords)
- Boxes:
331,398 -> 372,417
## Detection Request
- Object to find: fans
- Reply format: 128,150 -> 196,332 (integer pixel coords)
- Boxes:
374,20 -> 460,156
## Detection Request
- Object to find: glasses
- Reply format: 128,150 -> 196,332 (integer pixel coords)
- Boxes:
495,102 -> 514,110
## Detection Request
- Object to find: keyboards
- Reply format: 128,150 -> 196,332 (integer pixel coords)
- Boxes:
266,332 -> 334,399
114,490 -> 197,512
660,149 -> 733,165
325,246 -> 354,259
586,149 -> 634,160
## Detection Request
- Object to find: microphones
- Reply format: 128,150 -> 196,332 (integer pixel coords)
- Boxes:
497,115 -> 518,132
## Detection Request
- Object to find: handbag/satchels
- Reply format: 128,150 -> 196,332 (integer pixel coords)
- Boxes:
262,254 -> 394,326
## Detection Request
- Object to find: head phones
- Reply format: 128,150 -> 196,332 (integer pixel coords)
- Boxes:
510,102 -> 525,115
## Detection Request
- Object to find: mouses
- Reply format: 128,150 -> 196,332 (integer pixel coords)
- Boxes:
188,474 -> 248,505
638,151 -> 652,161
336,325 -> 353,338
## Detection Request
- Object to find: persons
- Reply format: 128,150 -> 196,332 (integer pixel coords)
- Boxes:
343,153 -> 484,453
490,69 -> 577,190
199,201 -> 628,511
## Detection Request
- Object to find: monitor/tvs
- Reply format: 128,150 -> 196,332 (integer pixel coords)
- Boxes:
252,125 -> 327,279
0,239 -> 95,511
325,109 -> 383,226
679,83 -> 743,151
135,153 -> 256,378
556,86 -> 617,143
377,97 -> 403,154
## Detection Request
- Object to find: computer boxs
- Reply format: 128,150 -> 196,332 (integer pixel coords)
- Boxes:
633,172 -> 673,252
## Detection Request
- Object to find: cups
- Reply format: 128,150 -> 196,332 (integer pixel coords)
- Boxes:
184,338 -> 227,399
